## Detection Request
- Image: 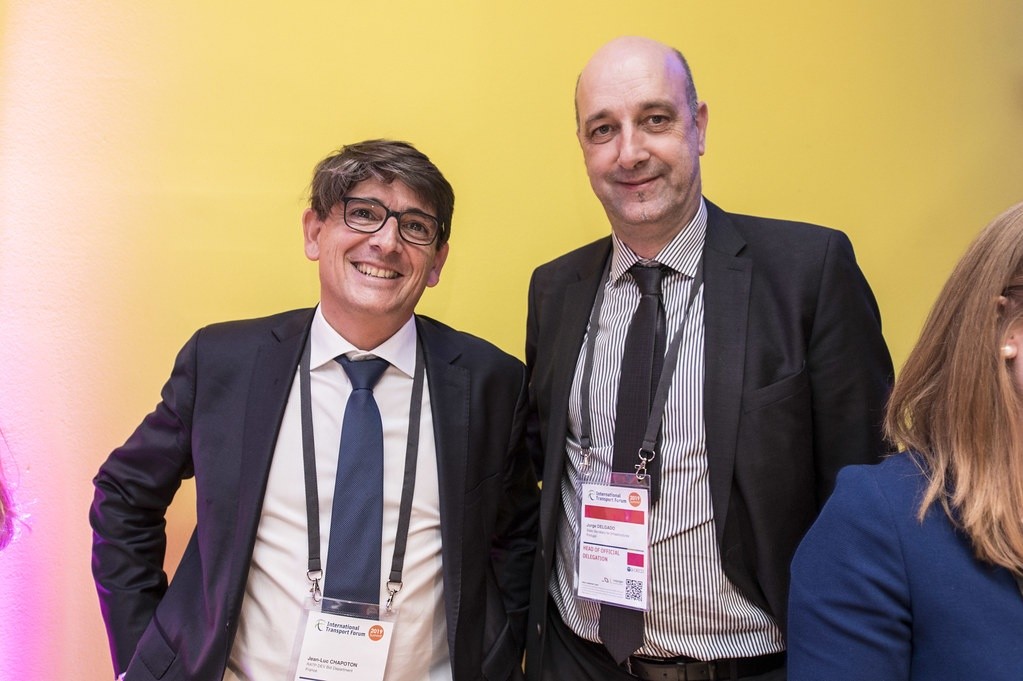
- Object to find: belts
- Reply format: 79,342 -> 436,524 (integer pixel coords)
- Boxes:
595,644 -> 787,681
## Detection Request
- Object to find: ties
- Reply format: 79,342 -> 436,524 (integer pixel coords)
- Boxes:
322,354 -> 390,620
598,261 -> 673,667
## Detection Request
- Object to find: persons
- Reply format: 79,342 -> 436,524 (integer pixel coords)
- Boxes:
486,36 -> 899,681
88,138 -> 530,681
786,202 -> 1023,681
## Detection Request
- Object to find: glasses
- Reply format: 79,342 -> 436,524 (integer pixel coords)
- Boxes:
339,196 -> 440,246
